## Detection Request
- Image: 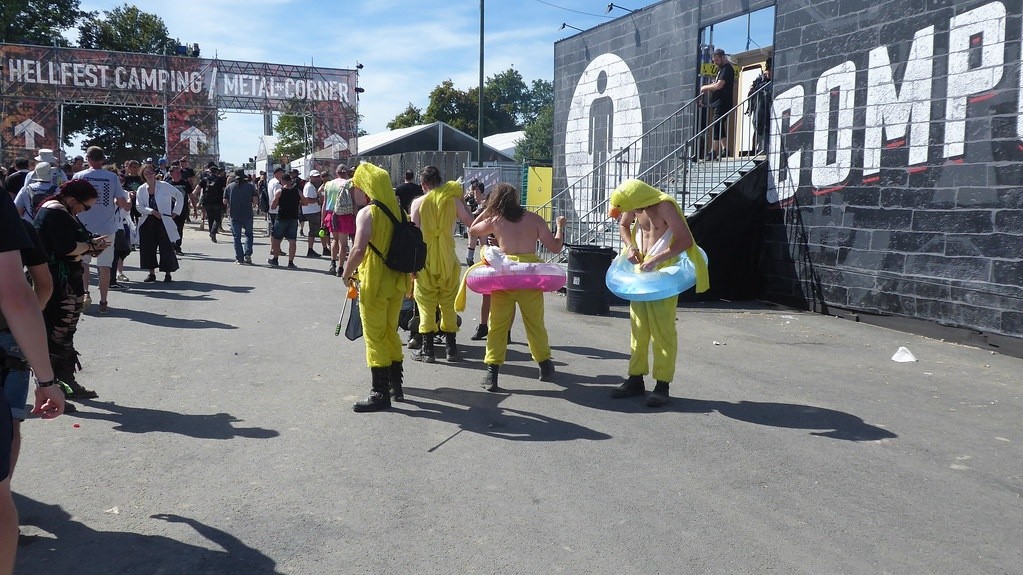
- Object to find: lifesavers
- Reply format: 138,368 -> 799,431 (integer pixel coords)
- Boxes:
605,245 -> 708,301
466,248 -> 566,294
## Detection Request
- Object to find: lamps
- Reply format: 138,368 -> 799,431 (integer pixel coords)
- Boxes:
605,3 -> 634,14
559,23 -> 584,33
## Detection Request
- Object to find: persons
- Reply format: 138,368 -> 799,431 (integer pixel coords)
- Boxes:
746,58 -> 767,156
399,297 -> 463,349
395,169 -> 425,213
410,166 -> 475,362
469,183 -> 567,391
701,49 -> 735,163
103,160 -> 146,290
71,146 -> 127,312
154,156 -> 369,277
136,164 -> 183,282
463,178 -> 511,344
0,186 -> 64,575
605,179 -> 709,405
342,161 -> 409,412
0,149 -> 90,287
32,180 -> 112,411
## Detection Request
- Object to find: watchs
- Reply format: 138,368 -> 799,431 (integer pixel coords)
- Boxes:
34,376 -> 58,388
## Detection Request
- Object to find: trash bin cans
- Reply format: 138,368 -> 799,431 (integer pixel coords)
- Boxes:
566,244 -> 614,315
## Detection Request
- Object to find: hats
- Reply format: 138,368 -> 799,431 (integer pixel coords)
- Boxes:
233,168 -> 249,179
310,170 -> 321,177
274,167 -> 283,174
86,146 -> 218,172
30,162 -> 54,182
34,149 -> 59,163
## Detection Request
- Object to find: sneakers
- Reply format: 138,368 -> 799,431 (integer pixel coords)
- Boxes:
471,324 -> 488,339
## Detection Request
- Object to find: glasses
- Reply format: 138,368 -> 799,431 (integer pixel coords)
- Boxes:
78,201 -> 92,212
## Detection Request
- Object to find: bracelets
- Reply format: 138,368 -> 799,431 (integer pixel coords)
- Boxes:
627,244 -> 633,251
468,247 -> 475,251
557,228 -> 563,232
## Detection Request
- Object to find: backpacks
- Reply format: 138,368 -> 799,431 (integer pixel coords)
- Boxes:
366,200 -> 428,273
201,178 -> 220,206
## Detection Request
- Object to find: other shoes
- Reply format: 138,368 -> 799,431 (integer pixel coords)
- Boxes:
64,402 -> 76,413
81,219 -> 346,315
57,374 -> 98,397
408,334 -> 422,348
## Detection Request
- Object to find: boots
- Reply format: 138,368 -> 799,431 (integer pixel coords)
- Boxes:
480,365 -> 500,391
613,374 -> 643,398
648,381 -> 669,406
539,359 -> 553,382
410,331 -> 435,364
446,333 -> 458,361
351,367 -> 390,412
390,360 -> 404,402
